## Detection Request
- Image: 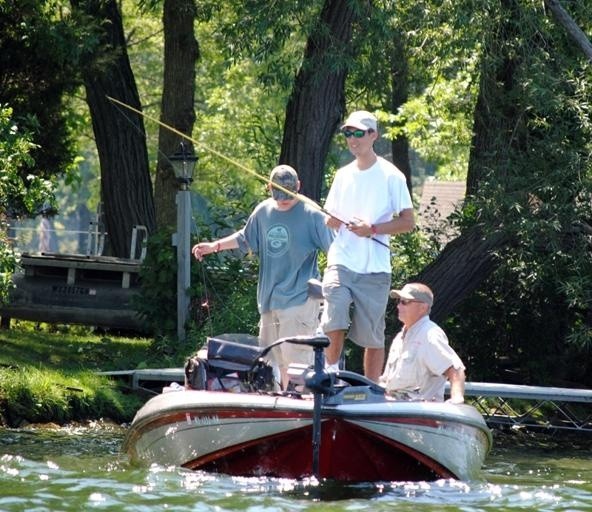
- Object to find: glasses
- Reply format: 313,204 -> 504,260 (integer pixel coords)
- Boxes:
401,299 -> 423,304
344,130 -> 364,138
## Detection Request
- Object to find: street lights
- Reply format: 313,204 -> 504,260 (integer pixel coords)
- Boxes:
168,134 -> 200,341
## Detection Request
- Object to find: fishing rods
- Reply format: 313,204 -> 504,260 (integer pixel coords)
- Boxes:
106,95 -> 392,249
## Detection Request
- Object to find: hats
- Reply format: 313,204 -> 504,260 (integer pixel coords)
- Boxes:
270,165 -> 298,200
340,111 -> 378,131
390,283 -> 433,305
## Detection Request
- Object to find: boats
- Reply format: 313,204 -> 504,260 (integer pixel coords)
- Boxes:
122,333 -> 496,487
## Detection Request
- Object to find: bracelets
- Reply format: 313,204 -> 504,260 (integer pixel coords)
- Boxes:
370,224 -> 378,237
215,240 -> 221,253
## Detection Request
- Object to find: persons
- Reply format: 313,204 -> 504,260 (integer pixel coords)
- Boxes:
40,210 -> 58,254
380,283 -> 466,406
190,164 -> 337,393
321,111 -> 416,383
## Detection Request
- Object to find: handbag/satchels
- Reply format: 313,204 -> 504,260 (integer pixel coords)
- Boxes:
184,355 -> 209,390
